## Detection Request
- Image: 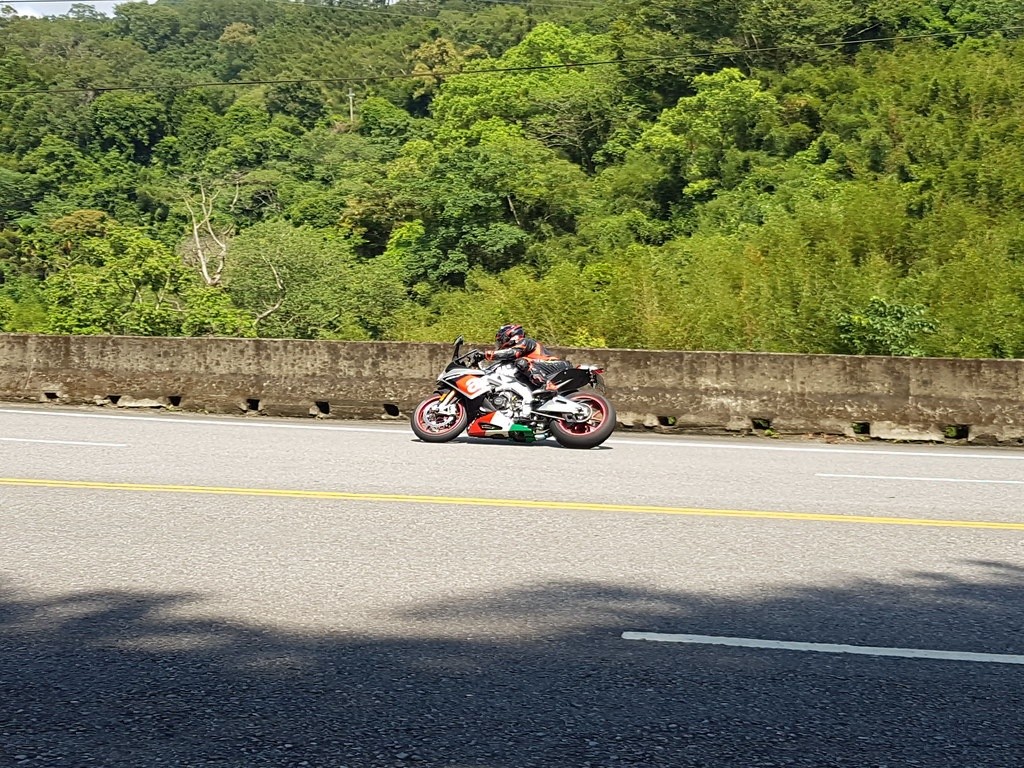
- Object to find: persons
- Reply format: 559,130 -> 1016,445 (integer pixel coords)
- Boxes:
473,325 -> 558,399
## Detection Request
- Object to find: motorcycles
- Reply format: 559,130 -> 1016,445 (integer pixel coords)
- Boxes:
409,335 -> 617,449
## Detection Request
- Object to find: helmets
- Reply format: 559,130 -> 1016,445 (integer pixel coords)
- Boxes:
495,325 -> 526,349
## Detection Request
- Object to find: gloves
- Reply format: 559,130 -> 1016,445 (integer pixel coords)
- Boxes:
474,352 -> 485,361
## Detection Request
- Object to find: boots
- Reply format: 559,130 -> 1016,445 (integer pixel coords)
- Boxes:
532,373 -> 558,397
